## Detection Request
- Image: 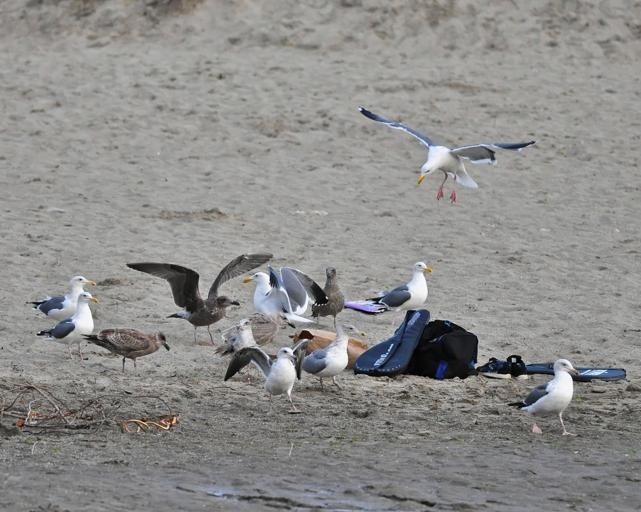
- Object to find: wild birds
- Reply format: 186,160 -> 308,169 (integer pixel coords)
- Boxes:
36,292 -> 100,365
26,275 -> 96,322
506,358 -> 579,437
213,264 -> 386,414
365,262 -> 433,324
356,106 -> 536,205
80,328 -> 170,373
126,252 -> 273,346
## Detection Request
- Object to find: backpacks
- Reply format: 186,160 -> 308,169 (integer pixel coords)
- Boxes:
406,319 -> 478,380
